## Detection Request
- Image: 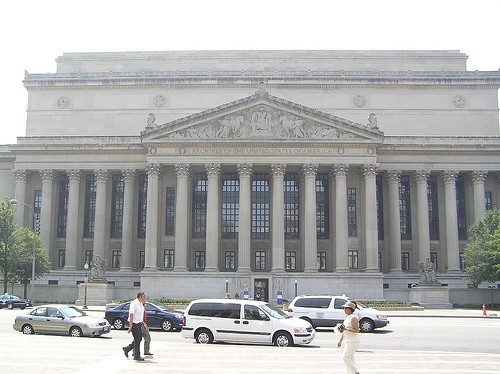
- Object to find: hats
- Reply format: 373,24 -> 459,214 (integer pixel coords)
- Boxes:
341,302 -> 355,310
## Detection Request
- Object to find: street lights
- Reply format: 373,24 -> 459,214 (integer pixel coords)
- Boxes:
81,262 -> 89,309
9,198 -> 37,307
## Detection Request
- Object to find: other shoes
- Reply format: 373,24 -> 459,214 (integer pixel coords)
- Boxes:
123,347 -> 128,357
144,354 -> 153,355
134,357 -> 144,361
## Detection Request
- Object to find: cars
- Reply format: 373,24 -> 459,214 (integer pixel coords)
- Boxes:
13,295 -> 32,307
13,304 -> 111,338
0,294 -> 26,310
103,300 -> 184,332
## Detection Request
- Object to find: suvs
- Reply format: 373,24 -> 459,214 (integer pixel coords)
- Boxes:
288,294 -> 390,333
180,299 -> 316,347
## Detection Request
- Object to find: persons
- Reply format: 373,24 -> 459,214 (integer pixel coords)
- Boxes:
337,302 -> 361,374
426,257 -> 434,281
94,255 -> 103,278
122,292 -> 147,361
132,300 -> 154,356
